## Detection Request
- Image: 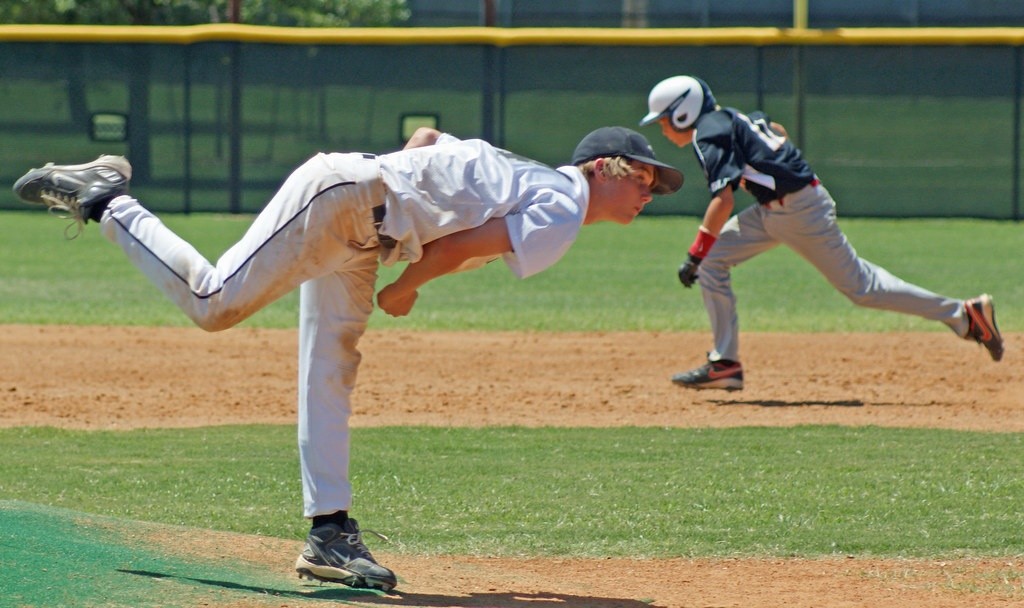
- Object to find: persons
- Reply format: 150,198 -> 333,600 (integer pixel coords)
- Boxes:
639,76 -> 1004,391
13,126 -> 683,592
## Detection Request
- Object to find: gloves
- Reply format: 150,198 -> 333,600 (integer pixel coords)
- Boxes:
679,252 -> 701,288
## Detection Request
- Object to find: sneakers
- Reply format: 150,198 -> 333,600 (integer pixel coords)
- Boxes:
296,519 -> 398,591
672,352 -> 744,391
963,293 -> 1004,361
11,154 -> 132,241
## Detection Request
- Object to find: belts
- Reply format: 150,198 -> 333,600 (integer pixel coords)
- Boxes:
362,154 -> 396,249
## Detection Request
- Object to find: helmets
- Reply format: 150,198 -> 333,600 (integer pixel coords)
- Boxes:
640,74 -> 716,132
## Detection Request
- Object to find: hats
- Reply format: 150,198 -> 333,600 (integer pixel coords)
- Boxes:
573,127 -> 683,195
